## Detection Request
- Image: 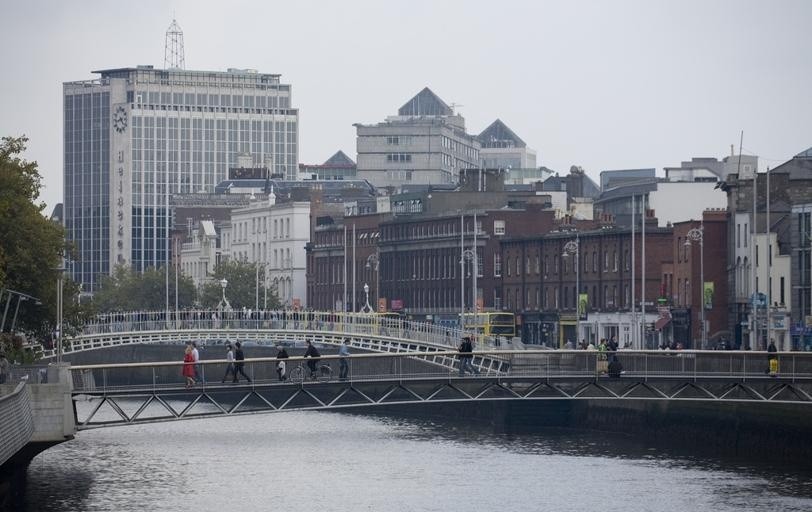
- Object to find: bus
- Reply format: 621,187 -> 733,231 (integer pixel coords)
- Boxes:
343,312 -> 404,336
282,311 -> 343,332
459,312 -> 516,342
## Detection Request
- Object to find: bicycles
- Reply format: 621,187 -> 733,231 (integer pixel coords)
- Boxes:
289,361 -> 333,385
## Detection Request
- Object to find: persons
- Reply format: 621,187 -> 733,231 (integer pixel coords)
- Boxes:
444,327 -> 451,345
459,337 -> 476,377
190,342 -> 206,385
75,307 -> 410,338
181,342 -> 196,388
233,342 -> 252,384
304,340 -> 320,377
339,339 -> 350,380
0,355 -> 8,384
222,345 -> 238,383
536,279 -> 811,379
275,346 -> 288,380
458,338 -> 472,376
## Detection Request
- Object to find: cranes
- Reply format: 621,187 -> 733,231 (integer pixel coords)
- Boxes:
448,102 -> 463,116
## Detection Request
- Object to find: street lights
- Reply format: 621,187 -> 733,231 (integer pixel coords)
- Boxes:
358,283 -> 374,313
49,265 -> 68,365
365,248 -> 380,312
683,220 -> 707,350
279,252 -> 294,309
0,286 -> 31,337
215,277 -> 233,311
9,293 -> 42,335
459,249 -> 477,342
561,234 -> 580,349
